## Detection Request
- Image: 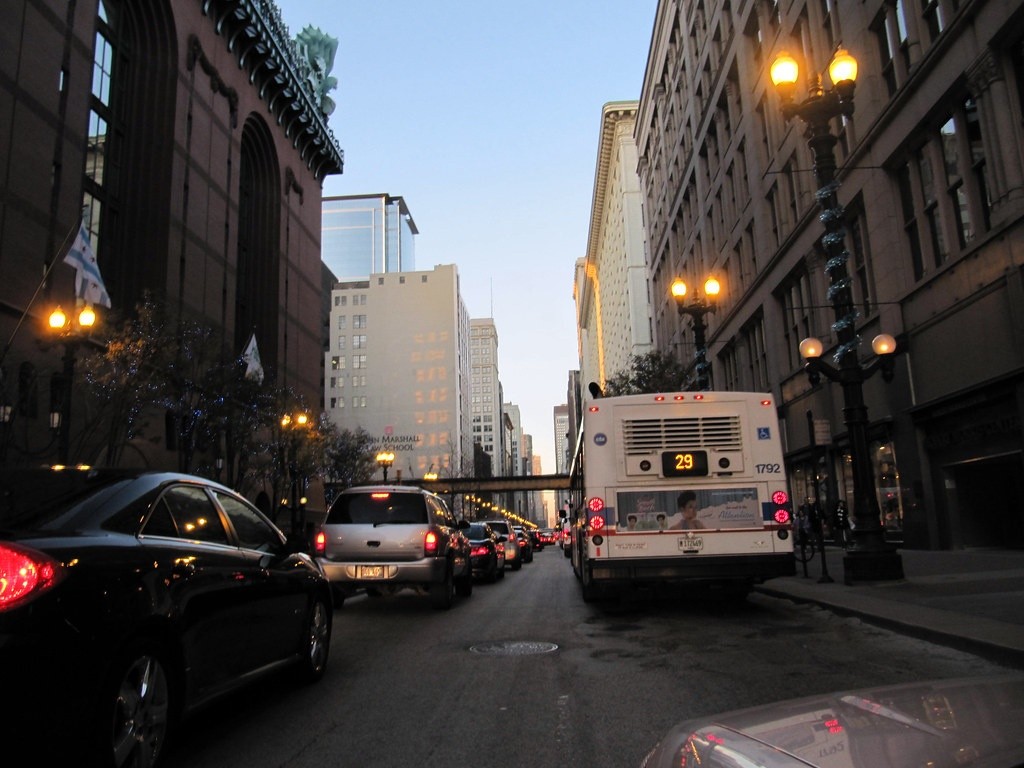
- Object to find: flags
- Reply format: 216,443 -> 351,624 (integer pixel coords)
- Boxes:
62,218 -> 112,309
241,331 -> 264,386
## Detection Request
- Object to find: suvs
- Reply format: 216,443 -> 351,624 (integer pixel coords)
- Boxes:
314,484 -> 475,602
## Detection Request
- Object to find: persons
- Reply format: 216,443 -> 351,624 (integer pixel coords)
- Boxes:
886,492 -> 899,529
628,515 -> 637,531
795,496 -> 854,551
656,514 -> 667,530
668,490 -> 707,530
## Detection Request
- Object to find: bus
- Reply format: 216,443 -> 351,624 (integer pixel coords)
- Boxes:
557,382 -> 795,607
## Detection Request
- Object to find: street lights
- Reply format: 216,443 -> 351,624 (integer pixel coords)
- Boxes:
768,46 -> 902,583
668,274 -> 722,390
277,411 -> 311,549
376,452 -> 395,485
464,494 -> 476,522
40,299 -> 95,466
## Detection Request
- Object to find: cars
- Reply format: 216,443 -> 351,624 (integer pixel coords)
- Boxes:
514,530 -> 535,564
460,521 -> 508,583
0,471 -> 335,768
479,520 -> 523,569
511,504 -> 573,551
